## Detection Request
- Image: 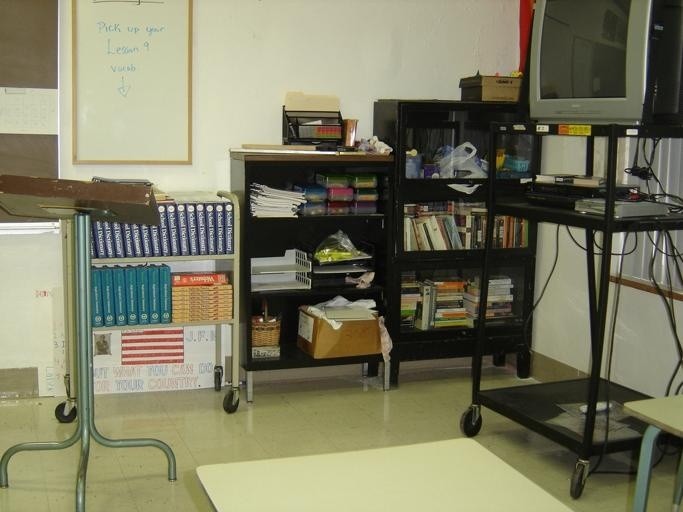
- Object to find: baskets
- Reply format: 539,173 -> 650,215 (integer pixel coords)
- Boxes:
251,297 -> 281,346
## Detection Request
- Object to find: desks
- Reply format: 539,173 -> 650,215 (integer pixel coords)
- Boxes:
191,434 -> 576,512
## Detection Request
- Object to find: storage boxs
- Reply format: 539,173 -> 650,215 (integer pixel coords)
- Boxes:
456,74 -> 525,101
296,303 -> 384,361
285,167 -> 378,215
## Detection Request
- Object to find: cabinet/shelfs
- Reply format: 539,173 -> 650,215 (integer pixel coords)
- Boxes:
452,108 -> 682,503
278,104 -> 351,152
53,189 -> 241,426
225,145 -> 399,405
373,95 -> 543,380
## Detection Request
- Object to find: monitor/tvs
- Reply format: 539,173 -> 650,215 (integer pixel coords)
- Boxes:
530,0 -> 683,125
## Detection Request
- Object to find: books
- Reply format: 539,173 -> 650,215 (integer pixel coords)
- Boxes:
89,187 -> 235,327
399,195 -> 526,331
535,173 -> 607,186
248,177 -> 305,220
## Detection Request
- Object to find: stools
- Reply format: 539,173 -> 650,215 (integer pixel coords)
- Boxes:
620,394 -> 681,512
